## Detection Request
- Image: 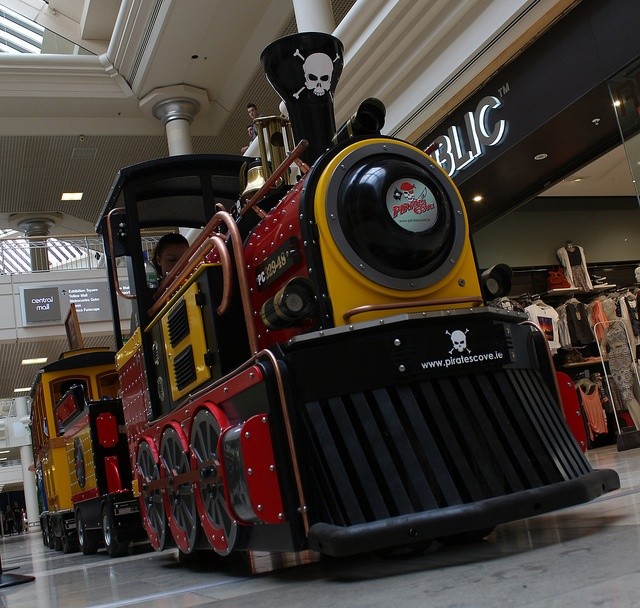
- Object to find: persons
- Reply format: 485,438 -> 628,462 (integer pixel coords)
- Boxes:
13,501 -> 23,535
247,102 -> 266,146
152,233 -> 190,280
600,298 -> 640,432
240,125 -> 254,152
556,240 -> 593,291
4,505 -> 15,536
634,267 -> 640,283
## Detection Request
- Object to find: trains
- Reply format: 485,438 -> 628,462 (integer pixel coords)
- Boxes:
30,32 -> 621,567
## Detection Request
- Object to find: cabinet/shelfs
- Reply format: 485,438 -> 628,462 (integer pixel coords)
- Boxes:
490,277 -> 639,444
